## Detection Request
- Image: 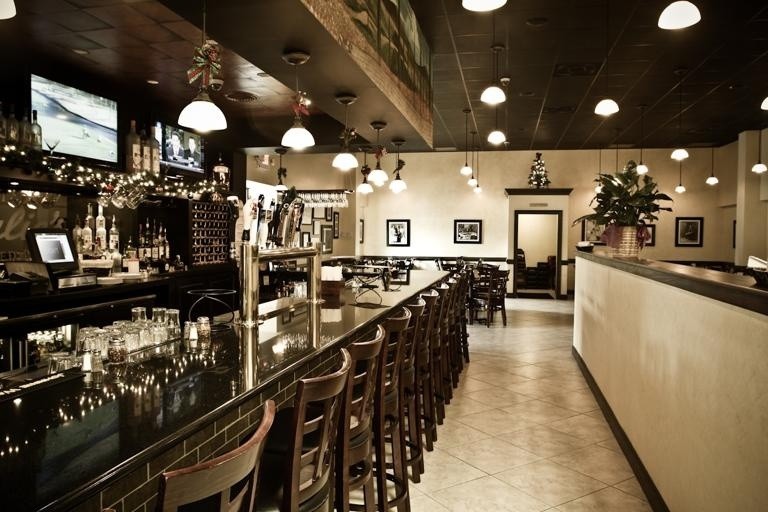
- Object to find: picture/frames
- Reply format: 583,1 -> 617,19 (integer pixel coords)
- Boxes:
734,220 -> 736,247
387,219 -> 410,247
453,219 -> 482,243
293,201 -> 339,253
675,217 -> 704,247
640,224 -> 655,245
359,219 -> 364,243
582,217 -> 606,245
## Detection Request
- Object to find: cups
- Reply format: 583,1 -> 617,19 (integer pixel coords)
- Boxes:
95,185 -> 148,209
81,307 -> 180,358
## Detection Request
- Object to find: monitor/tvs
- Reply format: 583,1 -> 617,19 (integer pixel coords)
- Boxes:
24,228 -> 79,269
161,118 -> 205,172
28,69 -> 128,173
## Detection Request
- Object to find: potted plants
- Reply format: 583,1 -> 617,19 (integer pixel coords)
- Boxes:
588,159 -> 673,255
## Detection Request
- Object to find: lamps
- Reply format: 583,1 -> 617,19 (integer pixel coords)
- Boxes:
333,95 -> 408,195
488,107 -> 506,148
176,1 -> 227,134
705,95 -> 768,186
278,53 -> 316,150
592,70 -> 689,196
658,1 -> 702,31
594,2 -> 619,117
481,14 -> 506,104
461,1 -> 508,14
461,110 -> 482,193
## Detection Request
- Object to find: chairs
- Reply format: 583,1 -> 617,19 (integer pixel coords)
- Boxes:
433,256 -> 509,328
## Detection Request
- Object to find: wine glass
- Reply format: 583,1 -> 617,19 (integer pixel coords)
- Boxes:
44,137 -> 61,155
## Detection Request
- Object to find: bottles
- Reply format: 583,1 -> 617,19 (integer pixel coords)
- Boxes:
108,219 -> 120,253
151,217 -> 158,261
72,217 -> 82,239
107,337 -> 129,363
96,219 -> 106,250
96,205 -> 106,228
127,120 -> 140,174
20,110 -> 32,143
124,234 -> 138,259
145,219 -> 152,257
85,202 -> 95,231
8,110 -> 20,144
82,219 -> 92,250
163,227 -> 171,259
183,320 -> 190,339
0,110 -> 8,139
80,348 -> 104,372
188,322 -> 199,339
197,317 -> 211,335
137,220 -> 145,258
158,221 -> 165,259
150,125 -> 159,175
31,110 -> 42,150
140,126 -> 150,173
